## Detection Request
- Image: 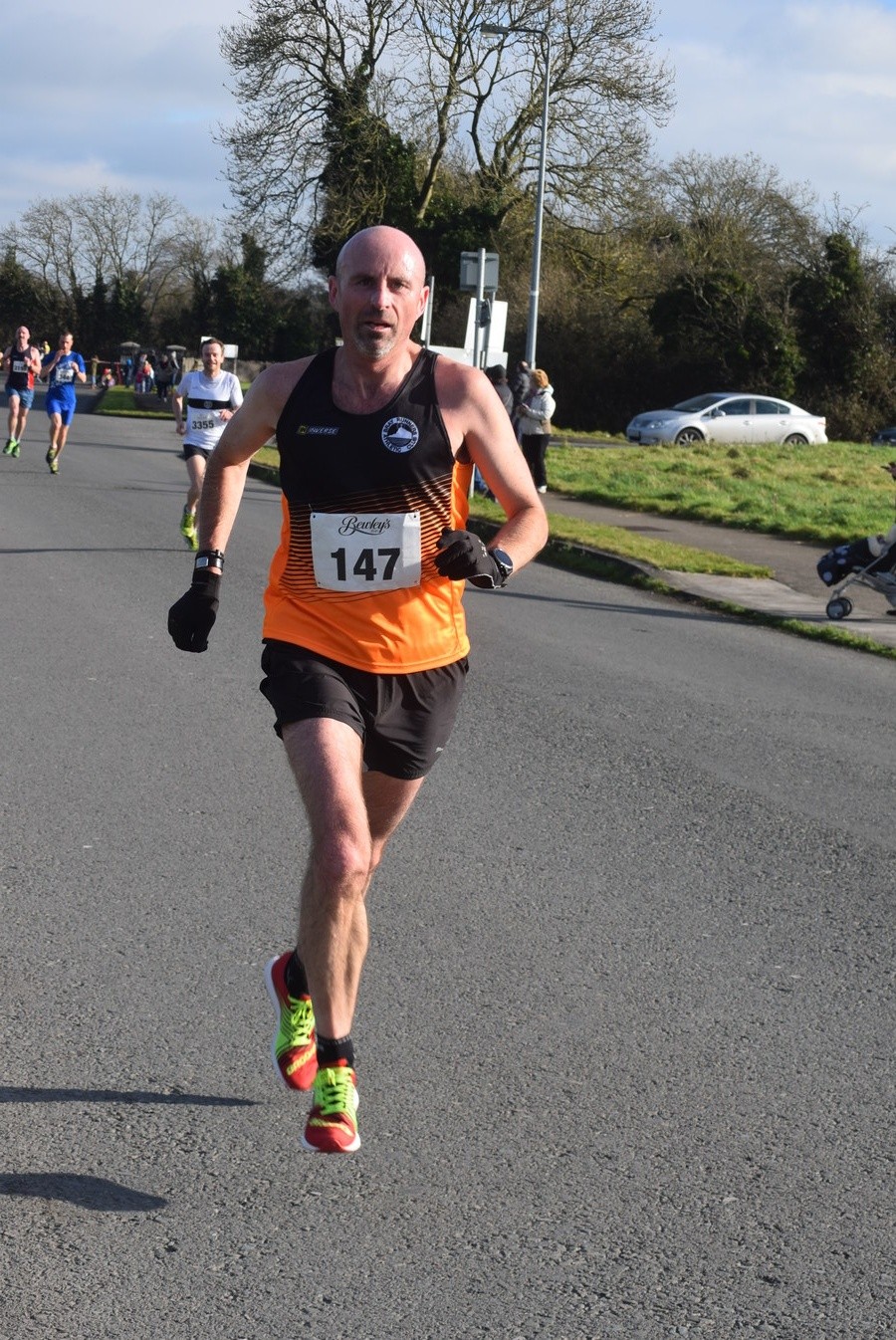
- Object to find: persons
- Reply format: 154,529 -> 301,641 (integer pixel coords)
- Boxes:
0,326 -> 42,458
172,338 -> 244,552
511,361 -> 533,427
484,365 -> 514,418
165,225 -> 551,1154
1,334 -> 202,404
515,368 -> 557,494
38,331 -> 87,474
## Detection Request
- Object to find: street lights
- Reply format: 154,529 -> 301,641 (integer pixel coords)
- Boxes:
478,24 -> 551,381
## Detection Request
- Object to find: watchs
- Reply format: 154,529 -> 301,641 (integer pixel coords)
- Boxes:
193,556 -> 224,572
487,548 -> 514,588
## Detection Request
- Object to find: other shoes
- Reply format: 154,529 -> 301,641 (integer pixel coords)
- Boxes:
539,485 -> 548,494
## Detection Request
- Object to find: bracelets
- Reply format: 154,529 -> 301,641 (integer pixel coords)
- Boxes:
194,549 -> 226,562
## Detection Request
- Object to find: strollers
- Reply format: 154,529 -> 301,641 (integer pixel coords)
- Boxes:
813,456 -> 896,622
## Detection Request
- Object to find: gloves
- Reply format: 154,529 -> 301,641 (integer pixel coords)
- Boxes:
168,567 -> 223,653
435,526 -> 503,589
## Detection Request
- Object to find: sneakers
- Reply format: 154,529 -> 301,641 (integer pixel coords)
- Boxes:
45,446 -> 57,462
3,438 -> 18,453
184,532 -> 199,552
181,505 -> 196,539
264,950 -> 319,1093
302,1059 -> 361,1155
50,462 -> 58,474
13,443 -> 21,457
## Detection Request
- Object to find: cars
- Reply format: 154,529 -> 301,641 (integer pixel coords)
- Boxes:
628,392 -> 830,455
